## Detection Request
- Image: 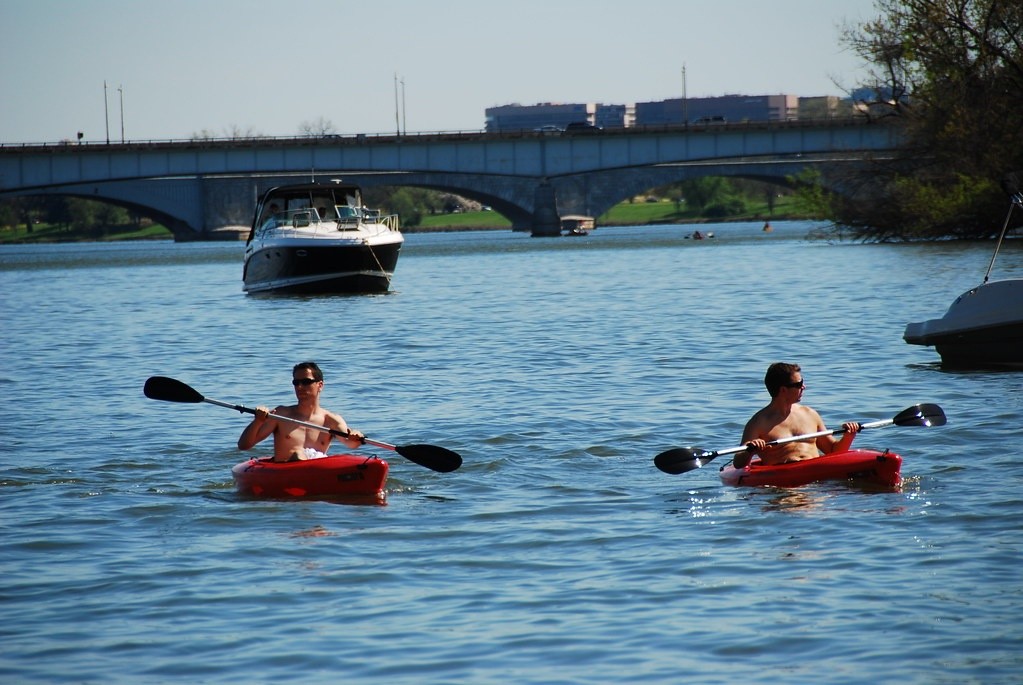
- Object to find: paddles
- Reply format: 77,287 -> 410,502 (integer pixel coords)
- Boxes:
141,373 -> 464,474
652,402 -> 949,476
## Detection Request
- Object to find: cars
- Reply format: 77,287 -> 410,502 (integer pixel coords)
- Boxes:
533,126 -> 566,137
322,135 -> 343,139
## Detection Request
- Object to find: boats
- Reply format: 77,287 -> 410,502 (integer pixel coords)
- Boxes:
241,183 -> 407,295
902,192 -> 1023,376
722,445 -> 903,494
231,453 -> 391,500
561,214 -> 595,236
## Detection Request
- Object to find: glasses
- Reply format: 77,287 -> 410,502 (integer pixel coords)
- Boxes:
783,378 -> 803,388
292,378 -> 318,386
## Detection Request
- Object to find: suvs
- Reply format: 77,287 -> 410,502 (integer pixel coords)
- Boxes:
681,115 -> 728,127
565,121 -> 603,132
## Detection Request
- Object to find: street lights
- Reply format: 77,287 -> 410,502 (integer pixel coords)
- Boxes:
117,84 -> 127,144
394,73 -> 406,135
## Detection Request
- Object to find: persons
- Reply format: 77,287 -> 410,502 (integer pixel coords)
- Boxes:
237,362 -> 365,463
695,231 -> 700,237
765,220 -> 769,228
264,204 -> 282,227
733,363 -> 859,469
318,207 -> 327,222
